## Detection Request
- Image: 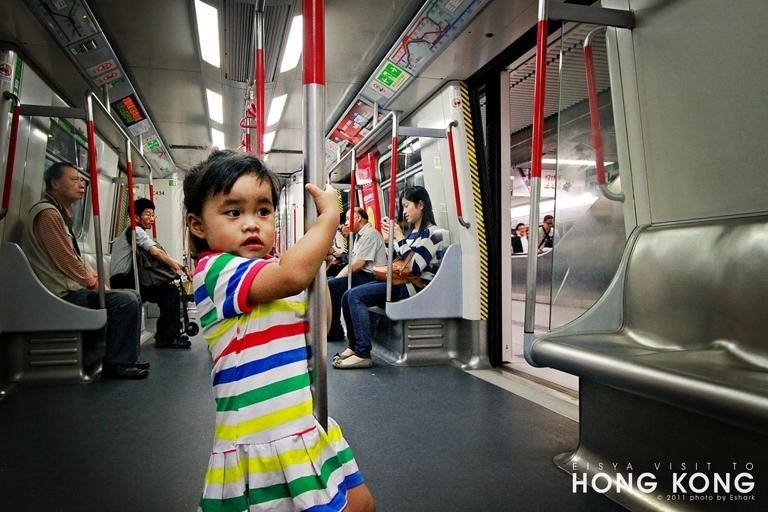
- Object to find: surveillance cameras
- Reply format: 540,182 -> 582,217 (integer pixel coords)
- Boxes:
484,32 -> 495,39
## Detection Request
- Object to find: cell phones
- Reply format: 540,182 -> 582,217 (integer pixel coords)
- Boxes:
381,216 -> 390,224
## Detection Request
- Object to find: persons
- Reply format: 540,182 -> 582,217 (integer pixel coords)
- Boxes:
510,222 -> 527,253
330,185 -> 444,370
108,197 -> 195,349
535,214 -> 560,255
182,149 -> 377,511
21,161 -> 152,380
328,205 -> 388,343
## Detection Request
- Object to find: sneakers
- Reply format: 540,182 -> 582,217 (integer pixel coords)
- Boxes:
333,345 -> 372,360
332,359 -> 372,369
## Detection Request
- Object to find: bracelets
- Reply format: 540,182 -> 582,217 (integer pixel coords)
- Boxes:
182,265 -> 188,272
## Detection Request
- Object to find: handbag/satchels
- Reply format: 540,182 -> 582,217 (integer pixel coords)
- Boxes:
373,261 -> 424,288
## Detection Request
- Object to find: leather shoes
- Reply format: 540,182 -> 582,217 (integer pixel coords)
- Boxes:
104,368 -> 148,380
156,339 -> 191,348
129,361 -> 150,369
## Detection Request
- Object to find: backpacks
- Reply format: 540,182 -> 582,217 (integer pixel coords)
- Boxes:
126,227 -> 177,288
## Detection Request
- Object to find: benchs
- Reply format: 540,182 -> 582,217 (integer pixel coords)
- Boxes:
329,242 -> 462,367
529,209 -> 768,511
0,239 -> 155,387
